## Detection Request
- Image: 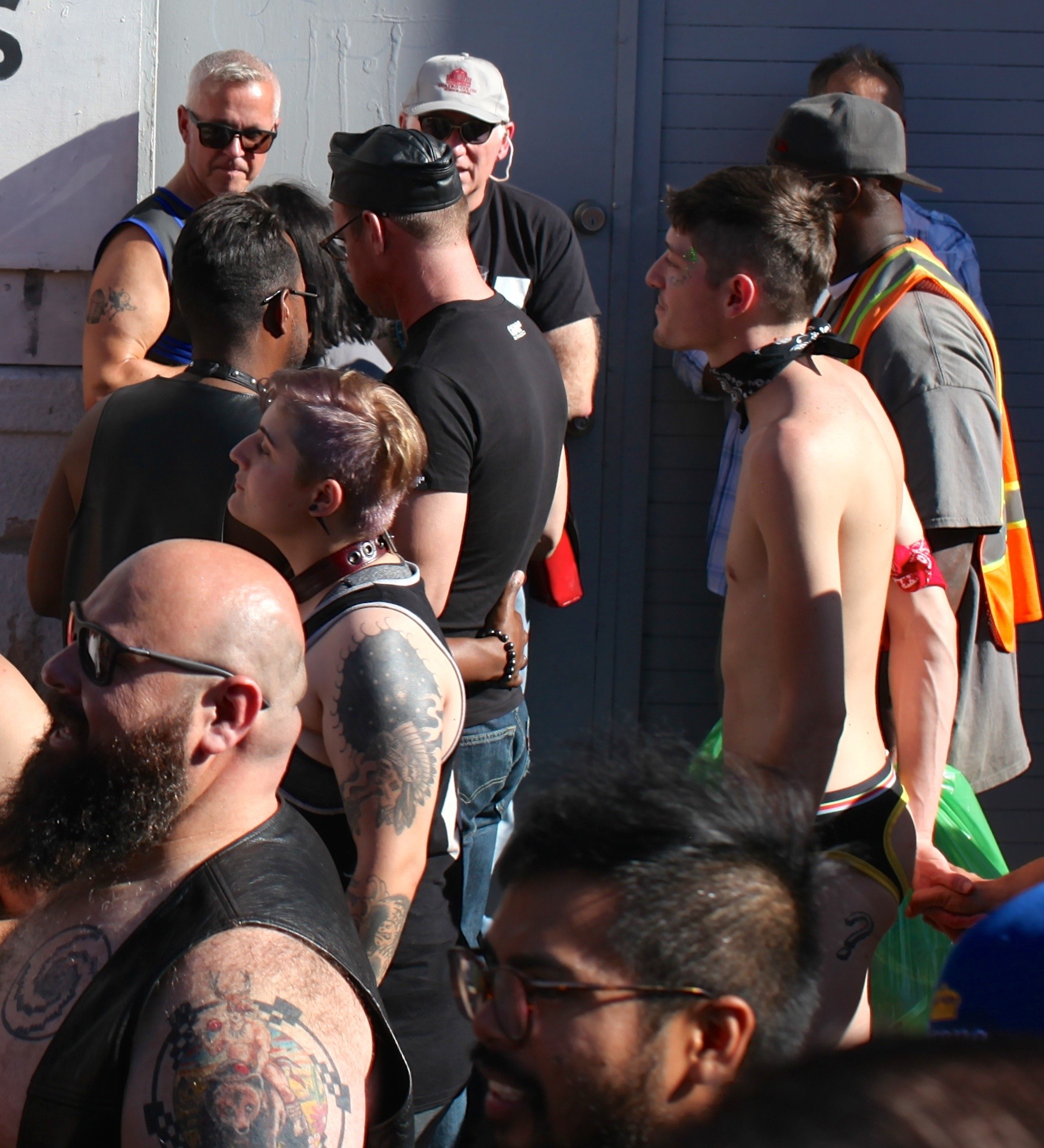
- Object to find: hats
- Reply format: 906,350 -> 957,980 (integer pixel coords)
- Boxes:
400,52 -> 510,123
327,124 -> 463,212
766,93 -> 944,194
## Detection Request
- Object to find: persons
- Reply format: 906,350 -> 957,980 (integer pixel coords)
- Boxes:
416,760 -> 1044,1148
228,367 -> 481,1148
0,538 -> 411,1148
646,46 -> 1044,1044
27,50 -> 602,948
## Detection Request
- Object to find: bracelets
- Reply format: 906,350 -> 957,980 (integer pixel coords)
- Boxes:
479,630 -> 516,682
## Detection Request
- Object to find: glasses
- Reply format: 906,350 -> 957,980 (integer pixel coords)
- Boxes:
414,115 -> 507,144
320,211 -> 390,262
445,945 -> 719,1048
263,288 -> 319,312
66,601 -> 270,710
185,109 -> 278,154
